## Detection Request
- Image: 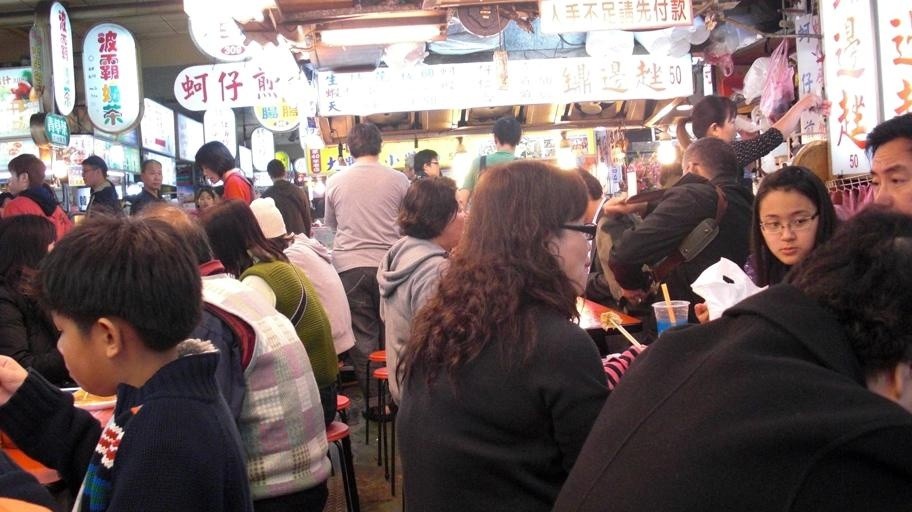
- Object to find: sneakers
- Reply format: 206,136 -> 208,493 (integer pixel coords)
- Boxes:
362,403 -> 398,423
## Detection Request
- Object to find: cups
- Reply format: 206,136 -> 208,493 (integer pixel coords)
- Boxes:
650,299 -> 691,338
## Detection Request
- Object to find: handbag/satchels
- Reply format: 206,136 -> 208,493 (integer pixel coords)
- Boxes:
596,198 -> 663,312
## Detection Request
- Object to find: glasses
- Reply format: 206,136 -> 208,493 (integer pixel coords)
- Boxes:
760,212 -> 819,233
558,224 -> 595,238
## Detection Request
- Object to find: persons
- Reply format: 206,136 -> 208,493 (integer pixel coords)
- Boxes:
0,143 -> 357,431
0,448 -> 60,511
0,214 -> 251,511
139,203 -> 333,511
570,95 -> 908,321
553,204 -> 911,512
396,157 -> 613,509
323,121 -> 441,420
460,118 -> 525,208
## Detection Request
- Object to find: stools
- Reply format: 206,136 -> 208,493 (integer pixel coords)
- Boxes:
325,347 -> 409,510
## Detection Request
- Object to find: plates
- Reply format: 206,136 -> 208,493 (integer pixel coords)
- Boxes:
70,400 -> 118,413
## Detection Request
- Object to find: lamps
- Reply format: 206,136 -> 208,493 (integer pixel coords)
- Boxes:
597,144 -> 627,176
487,0 -> 514,88
453,143 -> 473,173
557,138 -> 576,171
334,156 -> 347,170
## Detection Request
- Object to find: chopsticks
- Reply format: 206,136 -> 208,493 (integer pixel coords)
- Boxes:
609,319 -> 641,350
